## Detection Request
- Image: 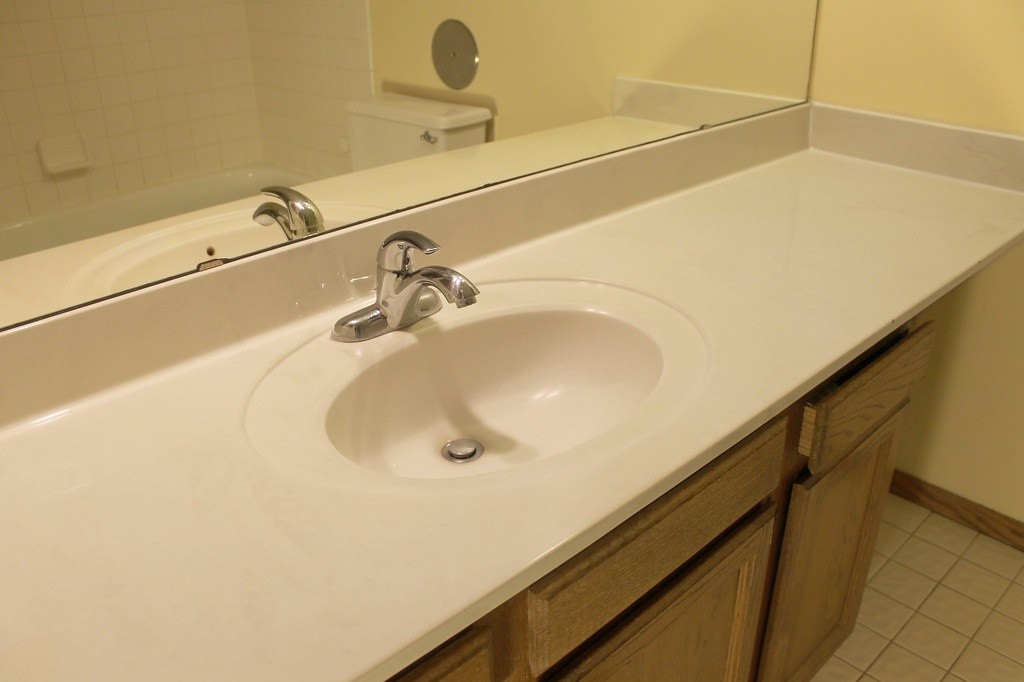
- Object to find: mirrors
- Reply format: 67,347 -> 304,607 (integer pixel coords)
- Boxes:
0,0 -> 821,333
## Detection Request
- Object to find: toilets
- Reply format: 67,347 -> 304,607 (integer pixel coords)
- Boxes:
346,91 -> 492,170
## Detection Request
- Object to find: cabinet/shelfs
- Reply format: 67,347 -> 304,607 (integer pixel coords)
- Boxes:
757,318 -> 956,682
393,413 -> 798,682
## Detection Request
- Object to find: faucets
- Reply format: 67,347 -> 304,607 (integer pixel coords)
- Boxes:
252,185 -> 327,241
330,229 -> 481,343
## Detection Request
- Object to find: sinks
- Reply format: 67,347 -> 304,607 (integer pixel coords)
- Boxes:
245,278 -> 708,499
0,164 -> 321,262
68,201 -> 393,300
